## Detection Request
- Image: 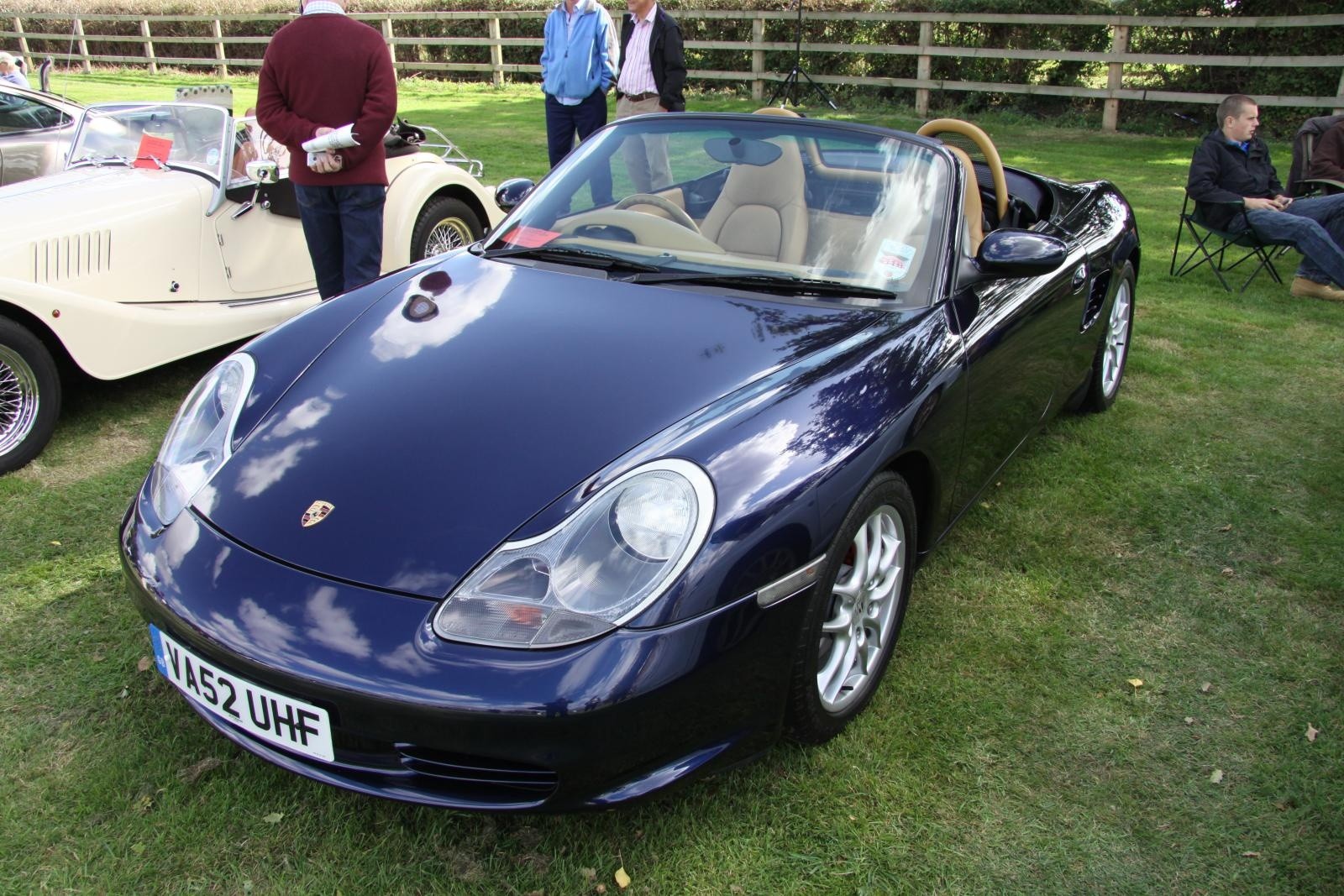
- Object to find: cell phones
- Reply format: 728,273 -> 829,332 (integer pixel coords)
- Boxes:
1276,198 -> 1293,210
307,152 -> 327,166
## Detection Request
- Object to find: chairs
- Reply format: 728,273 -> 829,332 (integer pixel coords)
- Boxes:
1273,132 -> 1344,260
40,56 -> 54,91
1171,118 -> 1284,291
701,106 -> 809,265
850,144 -> 984,287
230,140 -> 277,183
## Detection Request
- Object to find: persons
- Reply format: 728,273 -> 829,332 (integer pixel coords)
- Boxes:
614,0 -> 687,195
1310,120 -> 1344,195
1187,94 -> 1344,301
255,1 -> 397,301
0,51 -> 30,106
539,0 -> 620,218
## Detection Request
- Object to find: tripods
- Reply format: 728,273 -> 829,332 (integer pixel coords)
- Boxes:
766,0 -> 838,110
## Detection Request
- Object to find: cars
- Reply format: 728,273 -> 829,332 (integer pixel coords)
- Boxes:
1,78 -> 152,184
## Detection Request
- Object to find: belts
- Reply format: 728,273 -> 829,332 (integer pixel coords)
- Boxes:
624,93 -> 659,102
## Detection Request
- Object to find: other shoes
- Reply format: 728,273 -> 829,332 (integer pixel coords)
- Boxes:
1290,278 -> 1344,300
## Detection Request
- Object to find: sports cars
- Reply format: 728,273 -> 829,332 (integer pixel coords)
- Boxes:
117,104 -> 1155,811
1,100 -> 507,477
